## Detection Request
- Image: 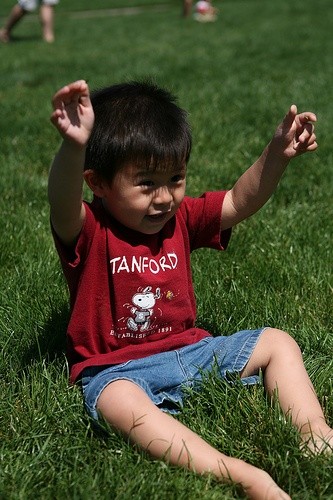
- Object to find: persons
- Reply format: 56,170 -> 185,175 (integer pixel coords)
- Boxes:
48,78 -> 333,500
0,0 -> 59,44
183,0 -> 213,17
